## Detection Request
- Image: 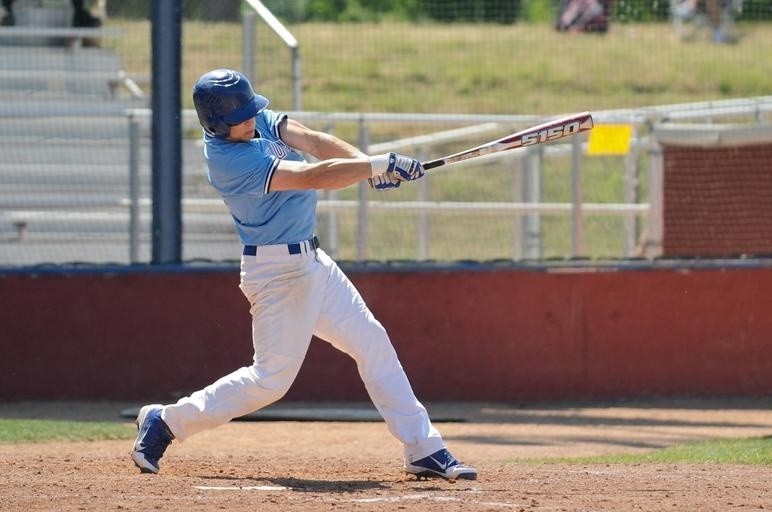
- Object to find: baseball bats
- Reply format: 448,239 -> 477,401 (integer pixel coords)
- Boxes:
369,111 -> 595,189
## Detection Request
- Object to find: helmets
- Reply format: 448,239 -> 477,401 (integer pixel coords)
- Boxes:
193,68 -> 270,140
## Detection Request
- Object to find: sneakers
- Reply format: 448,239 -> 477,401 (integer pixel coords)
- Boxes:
403,447 -> 478,481
130,404 -> 173,476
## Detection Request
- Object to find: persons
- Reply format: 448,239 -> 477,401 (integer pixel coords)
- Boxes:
555,1 -> 613,38
129,67 -> 479,483
661,0 -> 744,44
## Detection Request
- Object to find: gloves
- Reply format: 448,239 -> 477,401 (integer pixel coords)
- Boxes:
368,151 -> 424,182
368,174 -> 402,191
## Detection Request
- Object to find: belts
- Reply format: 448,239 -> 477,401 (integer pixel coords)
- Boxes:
243,235 -> 321,256
